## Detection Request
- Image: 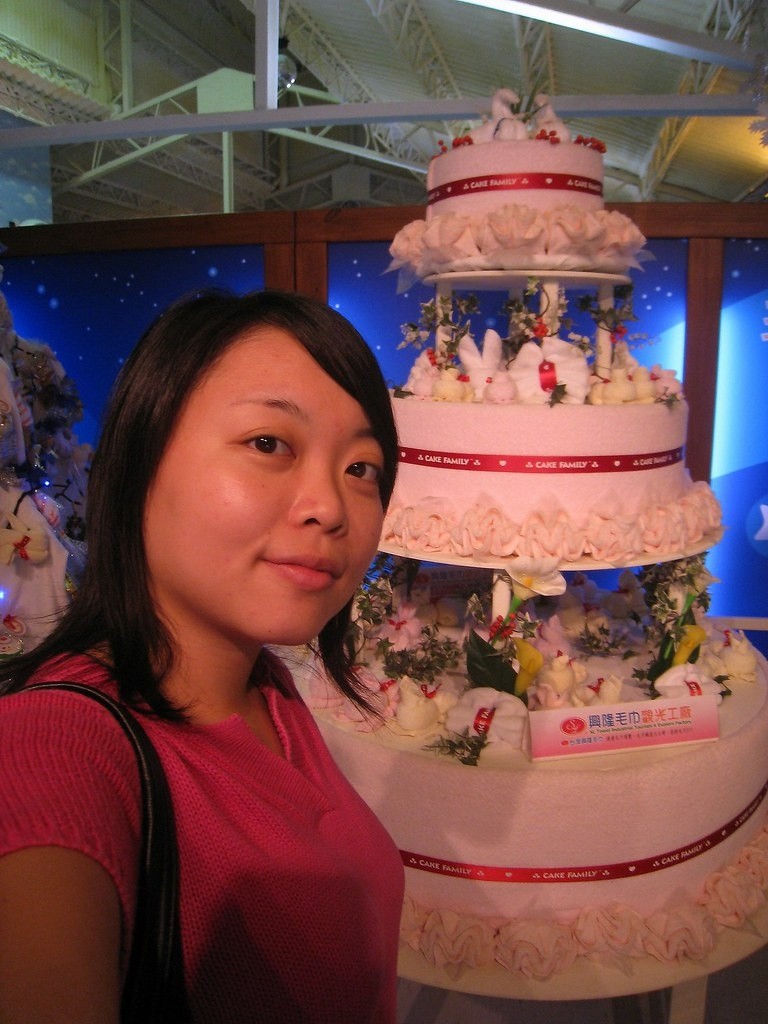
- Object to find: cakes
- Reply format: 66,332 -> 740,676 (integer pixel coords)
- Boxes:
266,88 -> 768,979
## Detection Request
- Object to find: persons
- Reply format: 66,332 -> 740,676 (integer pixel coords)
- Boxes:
0,286 -> 406,1024
